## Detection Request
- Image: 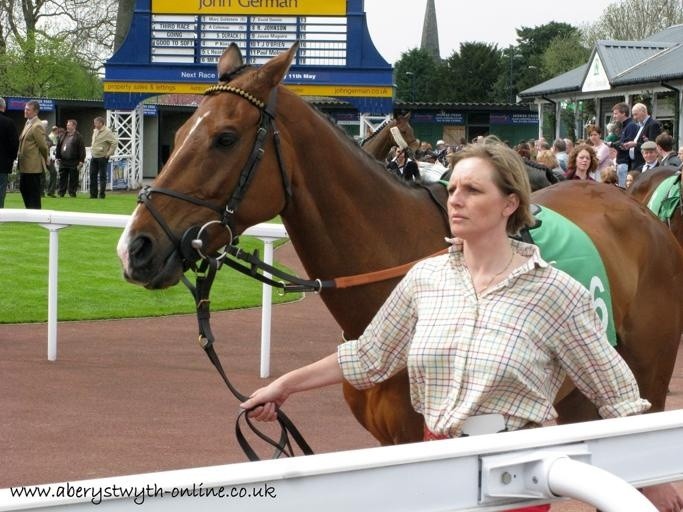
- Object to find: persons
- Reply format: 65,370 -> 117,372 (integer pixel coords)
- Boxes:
238,133 -> 683,511
41,119 -> 58,199
88,116 -> 118,199
0,97 -> 20,208
384,101 -> 683,185
55,119 -> 86,198
47,125 -> 64,151
16,100 -> 49,209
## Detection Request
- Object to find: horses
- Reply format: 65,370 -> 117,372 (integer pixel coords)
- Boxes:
114,38 -> 683,448
626,161 -> 683,251
357,109 -> 418,164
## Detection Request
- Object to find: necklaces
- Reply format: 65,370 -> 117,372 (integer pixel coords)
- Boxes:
475,248 -> 515,298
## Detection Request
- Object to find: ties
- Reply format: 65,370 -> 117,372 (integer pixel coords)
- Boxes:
645,165 -> 649,170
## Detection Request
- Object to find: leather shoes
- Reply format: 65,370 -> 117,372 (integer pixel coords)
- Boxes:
91,194 -> 97,198
99,193 -> 104,198
41,191 -> 76,198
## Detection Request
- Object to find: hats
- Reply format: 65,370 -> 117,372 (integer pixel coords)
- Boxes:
640,141 -> 656,150
